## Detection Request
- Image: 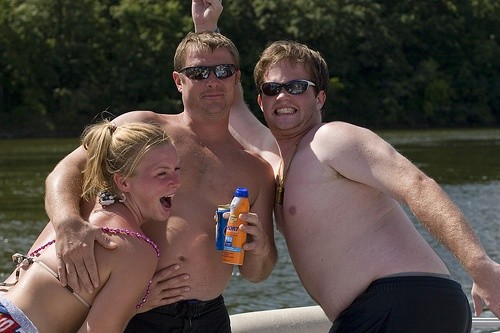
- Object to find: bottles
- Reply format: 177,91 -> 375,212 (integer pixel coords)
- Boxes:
222,187 -> 250,265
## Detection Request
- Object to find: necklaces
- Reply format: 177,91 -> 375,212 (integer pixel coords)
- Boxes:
274,131 -> 311,209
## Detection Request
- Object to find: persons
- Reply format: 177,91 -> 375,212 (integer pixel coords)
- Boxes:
44,32 -> 285,332
1,122 -> 190,333
191,1 -> 500,333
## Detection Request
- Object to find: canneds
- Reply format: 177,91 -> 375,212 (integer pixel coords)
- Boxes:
215,204 -> 231,250
